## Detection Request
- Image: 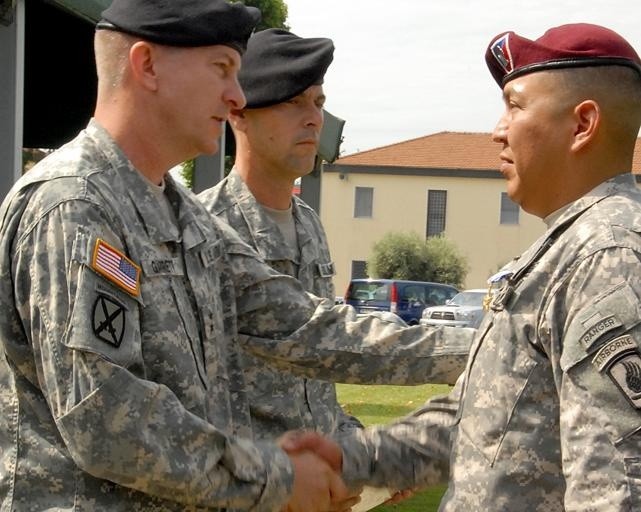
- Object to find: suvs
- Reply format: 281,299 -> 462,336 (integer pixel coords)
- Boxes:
418,287 -> 495,332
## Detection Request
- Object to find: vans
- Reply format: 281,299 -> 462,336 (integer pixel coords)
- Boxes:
343,277 -> 461,330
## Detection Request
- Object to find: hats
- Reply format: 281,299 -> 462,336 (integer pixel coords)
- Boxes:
232,29 -> 335,109
94,1 -> 263,56
484,21 -> 641,91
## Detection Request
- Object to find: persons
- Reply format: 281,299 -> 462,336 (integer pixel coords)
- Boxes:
192,26 -> 438,506
284,24 -> 641,511
0,1 -> 480,511
